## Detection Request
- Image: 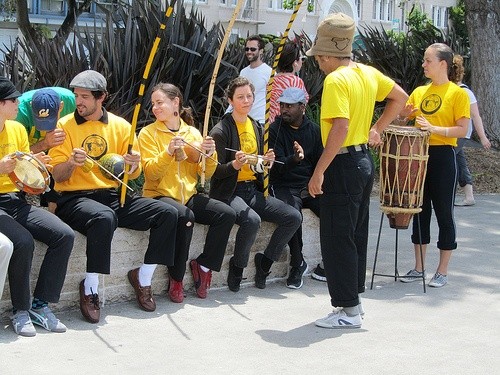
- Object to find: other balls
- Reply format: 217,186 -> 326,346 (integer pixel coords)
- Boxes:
99,153 -> 124,181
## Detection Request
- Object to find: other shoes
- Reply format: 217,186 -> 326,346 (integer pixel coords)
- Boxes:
314,308 -> 365,329
428,271 -> 448,288
399,267 -> 427,282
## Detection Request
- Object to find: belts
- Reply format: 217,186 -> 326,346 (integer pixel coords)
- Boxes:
336,142 -> 368,155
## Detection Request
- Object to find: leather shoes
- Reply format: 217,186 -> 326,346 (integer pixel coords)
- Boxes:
127,266 -> 156,312
79,278 -> 101,323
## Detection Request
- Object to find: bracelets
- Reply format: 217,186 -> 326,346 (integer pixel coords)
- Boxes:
445,127 -> 448,137
166,146 -> 173,156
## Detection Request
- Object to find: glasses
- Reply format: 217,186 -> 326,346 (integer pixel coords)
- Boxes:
4,97 -> 16,103
244,47 -> 256,52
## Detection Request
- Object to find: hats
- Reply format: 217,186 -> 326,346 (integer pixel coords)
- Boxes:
69,69 -> 107,92
305,13 -> 355,57
0,76 -> 21,99
32,89 -> 60,131
276,87 -> 306,104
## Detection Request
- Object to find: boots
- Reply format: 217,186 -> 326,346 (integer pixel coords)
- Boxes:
454,184 -> 477,206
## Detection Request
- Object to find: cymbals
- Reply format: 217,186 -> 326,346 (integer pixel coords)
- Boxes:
8,152 -> 51,195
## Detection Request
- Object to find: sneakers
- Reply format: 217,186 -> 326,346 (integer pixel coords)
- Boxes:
228,257 -> 248,292
168,270 -> 184,302
311,264 -> 327,281
29,306 -> 67,333
10,309 -> 36,338
286,258 -> 308,289
255,252 -> 272,289
190,259 -> 212,298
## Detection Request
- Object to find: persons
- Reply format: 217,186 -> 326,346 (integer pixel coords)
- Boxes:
302,12 -> 409,329
14,86 -> 77,207
0,77 -> 74,336
49,70 -> 178,324
206,76 -> 300,292
452,69 -> 492,206
221,36 -> 276,129
402,43 -> 468,289
269,45 -> 309,127
137,83 -> 235,302
268,87 -> 328,289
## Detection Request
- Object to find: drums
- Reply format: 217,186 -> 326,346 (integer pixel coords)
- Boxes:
379,125 -> 430,228
9,153 -> 50,195
